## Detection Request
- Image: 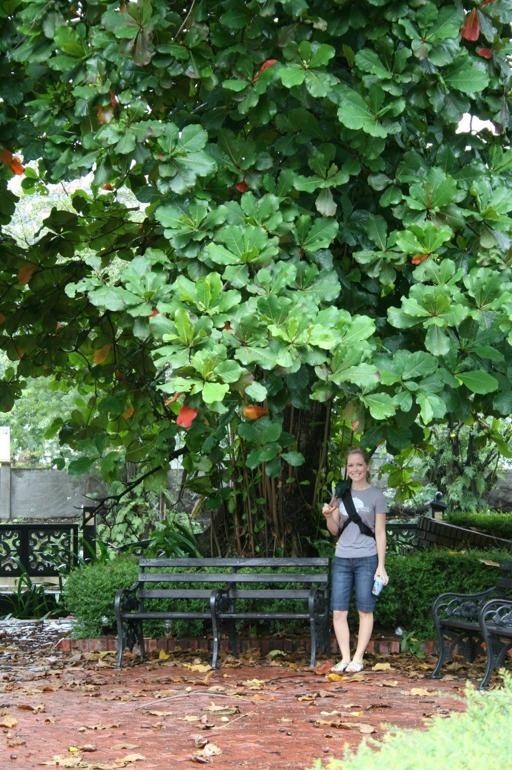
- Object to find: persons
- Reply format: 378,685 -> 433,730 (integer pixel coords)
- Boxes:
322,448 -> 390,674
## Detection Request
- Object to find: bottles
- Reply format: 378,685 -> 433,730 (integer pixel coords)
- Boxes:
370,572 -> 385,596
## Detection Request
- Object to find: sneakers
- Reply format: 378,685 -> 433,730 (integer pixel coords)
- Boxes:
346,662 -> 364,673
331,660 -> 351,672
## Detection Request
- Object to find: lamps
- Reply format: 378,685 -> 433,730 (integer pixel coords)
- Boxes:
83,504 -> 98,565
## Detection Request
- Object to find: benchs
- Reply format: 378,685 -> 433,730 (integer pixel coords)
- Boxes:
0,521 -> 80,601
430,562 -> 511,690
111,556 -> 334,668
